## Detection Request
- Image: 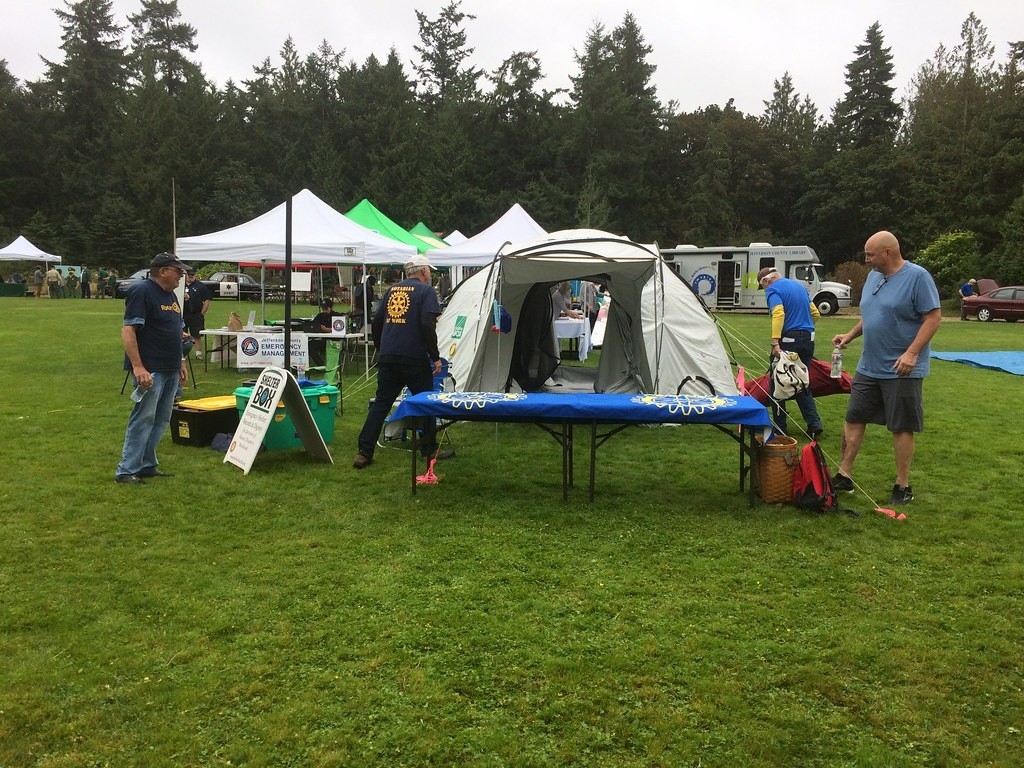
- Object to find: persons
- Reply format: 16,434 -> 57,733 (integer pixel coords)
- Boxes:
831,231 -> 942,506
351,275 -> 377,333
756,267 -> 823,438
114,252 -> 192,486
352,255 -> 455,469
174,268 -> 211,399
552,281 -> 610,350
436,271 -> 451,307
0,264 -> 130,299
309,298 -> 344,366
959,278 -> 978,321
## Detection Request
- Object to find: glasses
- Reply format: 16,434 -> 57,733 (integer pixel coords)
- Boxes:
872,279 -> 886,296
167,267 -> 183,275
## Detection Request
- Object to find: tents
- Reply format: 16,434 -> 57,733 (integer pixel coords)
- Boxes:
393,229 -> 739,426
174,189 -> 549,381
0,234 -> 62,298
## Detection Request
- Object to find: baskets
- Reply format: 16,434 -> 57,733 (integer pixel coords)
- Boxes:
753,435 -> 799,505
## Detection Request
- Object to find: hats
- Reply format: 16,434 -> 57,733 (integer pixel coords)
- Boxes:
368,275 -> 377,286
186,266 -> 197,275
320,298 -> 333,308
404,254 -> 438,270
149,252 -> 194,271
756,268 -> 777,291
68,267 -> 75,273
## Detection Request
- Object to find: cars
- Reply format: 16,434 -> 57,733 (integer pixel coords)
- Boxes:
962,278 -> 1024,322
115,268 -> 152,299
199,272 -> 271,301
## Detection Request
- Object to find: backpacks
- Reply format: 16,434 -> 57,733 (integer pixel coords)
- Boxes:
795,440 -> 836,513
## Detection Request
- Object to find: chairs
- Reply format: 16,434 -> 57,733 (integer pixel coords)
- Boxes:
350,324 -> 378,363
248,291 -> 343,304
290,338 -> 344,418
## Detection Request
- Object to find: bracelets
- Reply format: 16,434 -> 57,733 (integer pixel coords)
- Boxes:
771,343 -> 779,348
181,357 -> 186,361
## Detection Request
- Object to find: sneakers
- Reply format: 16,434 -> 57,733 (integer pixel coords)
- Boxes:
832,473 -> 855,494
885,483 -> 914,505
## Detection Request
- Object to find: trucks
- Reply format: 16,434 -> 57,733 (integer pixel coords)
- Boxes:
660,240 -> 853,317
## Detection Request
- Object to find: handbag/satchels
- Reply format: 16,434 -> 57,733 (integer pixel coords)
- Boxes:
228,312 -> 242,331
772,349 -> 810,402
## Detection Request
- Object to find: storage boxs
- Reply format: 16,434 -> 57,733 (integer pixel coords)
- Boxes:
170,396 -> 239,448
233,383 -> 340,450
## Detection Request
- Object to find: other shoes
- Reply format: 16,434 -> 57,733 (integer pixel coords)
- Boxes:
112,474 -> 149,486
353,456 -> 375,468
138,468 -> 174,478
803,425 -> 823,436
961,317 -> 970,321
195,355 -> 204,362
421,447 -> 455,463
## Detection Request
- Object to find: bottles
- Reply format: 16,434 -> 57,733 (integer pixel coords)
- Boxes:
129,372 -> 156,402
830,343 -> 842,378
297,357 -> 306,380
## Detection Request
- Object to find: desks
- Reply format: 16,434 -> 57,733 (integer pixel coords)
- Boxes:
407,391 -> 767,503
553,309 -> 586,362
199,329 -> 364,378
